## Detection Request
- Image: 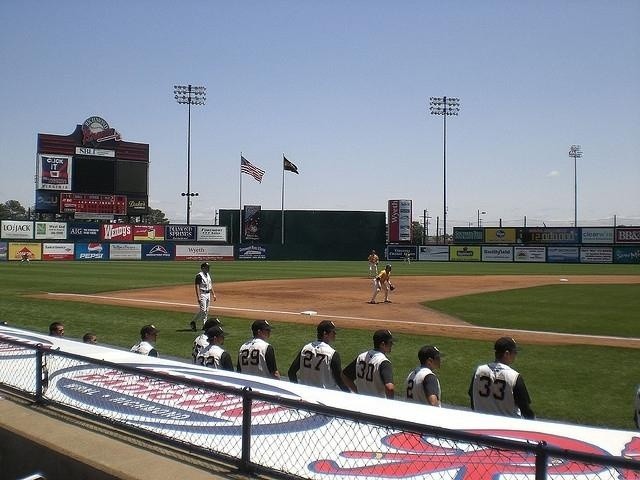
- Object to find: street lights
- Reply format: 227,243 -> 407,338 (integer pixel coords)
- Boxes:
430,95 -> 461,247
173,81 -> 207,226
569,142 -> 584,229
477,209 -> 487,226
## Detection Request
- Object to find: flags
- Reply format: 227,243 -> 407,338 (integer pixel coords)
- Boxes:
284,157 -> 299,175
240,156 -> 265,184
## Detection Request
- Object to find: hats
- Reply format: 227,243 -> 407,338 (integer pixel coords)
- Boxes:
252,319 -> 276,330
205,318 -> 229,338
418,345 -> 446,360
374,329 -> 398,342
142,325 -> 159,335
494,337 -> 522,352
202,262 -> 211,267
316,320 -> 340,332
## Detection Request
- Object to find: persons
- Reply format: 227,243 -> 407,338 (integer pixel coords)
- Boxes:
191,318 -> 281,379
83,333 -> 97,345
81,254 -> 96,265
342,329 -> 395,400
468,337 -> 535,420
404,345 -> 448,408
289,320 -> 351,393
130,325 -> 160,359
371,264 -> 393,304
191,262 -> 216,332
49,322 -> 64,338
404,250 -> 410,264
20,252 -> 29,264
368,250 -> 379,278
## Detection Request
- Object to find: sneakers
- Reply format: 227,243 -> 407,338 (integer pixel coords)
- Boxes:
189,321 -> 196,331
